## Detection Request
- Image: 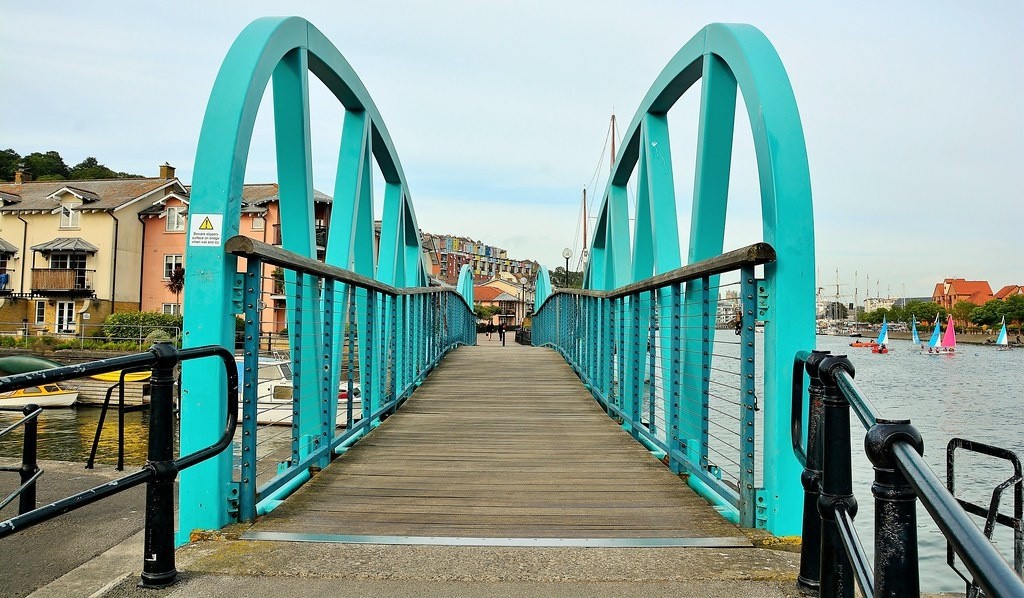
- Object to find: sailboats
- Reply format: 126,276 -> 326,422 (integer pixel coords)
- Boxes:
917,313 -> 951,354
872,313 -> 896,354
911,314 -> 925,348
994,320 -> 1013,351
941,314 -> 964,354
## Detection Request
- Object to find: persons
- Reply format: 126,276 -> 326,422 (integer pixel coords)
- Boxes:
986,338 -> 996,343
497,320 -> 504,341
486,320 -> 494,341
878,343 -> 885,353
943,347 -> 947,351
936,347 -> 940,353
871,339 -> 874,343
948,347 -> 954,352
928,347 -> 933,353
1016,335 -> 1021,344
1001,345 -> 1011,349
856,340 -> 859,343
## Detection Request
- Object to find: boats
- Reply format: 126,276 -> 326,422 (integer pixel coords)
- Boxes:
849,342 -> 879,346
983,340 -> 996,345
234,356 -> 361,427
850,332 -> 861,337
1013,344 -> 1024,347
0,356 -> 79,409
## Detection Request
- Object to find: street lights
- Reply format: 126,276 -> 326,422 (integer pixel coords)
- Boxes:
562,248 -> 573,288
520,277 -> 527,331
